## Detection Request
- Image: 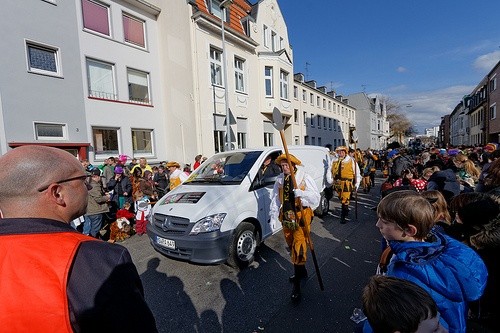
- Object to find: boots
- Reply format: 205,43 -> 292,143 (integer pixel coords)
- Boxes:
341,208 -> 346,224
291,263 -> 304,300
289,266 -> 308,282
345,208 -> 348,216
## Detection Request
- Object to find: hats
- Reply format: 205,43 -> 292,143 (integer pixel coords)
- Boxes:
91,169 -> 99,175
484,144 -> 494,150
115,167 -> 123,174
166,162 -> 180,168
275,153 -> 301,165
335,146 -> 348,153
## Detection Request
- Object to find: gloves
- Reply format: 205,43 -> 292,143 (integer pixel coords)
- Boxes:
292,188 -> 305,199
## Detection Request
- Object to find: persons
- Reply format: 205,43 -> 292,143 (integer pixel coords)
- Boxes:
422,166 -> 461,200
476,150 -> 500,192
106,166 -> 134,230
330,146 -> 361,223
449,192 -> 500,264
154,165 -> 169,198
484,157 -> 500,199
116,202 -> 135,220
362,274 -> 448,333
453,153 -> 482,190
420,190 -> 452,236
362,190 -> 488,333
0,145 -> 157,333
275,152 -> 313,303
392,165 -> 426,193
0,140 -> 500,333
166,162 -> 188,190
131,157 -> 152,177
115,153 -> 129,175
83,170 -> 109,238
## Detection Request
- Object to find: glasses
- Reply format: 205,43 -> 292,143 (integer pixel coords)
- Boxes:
38,175 -> 91,192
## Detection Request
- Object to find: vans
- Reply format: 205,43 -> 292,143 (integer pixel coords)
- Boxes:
145,144 -> 333,268
